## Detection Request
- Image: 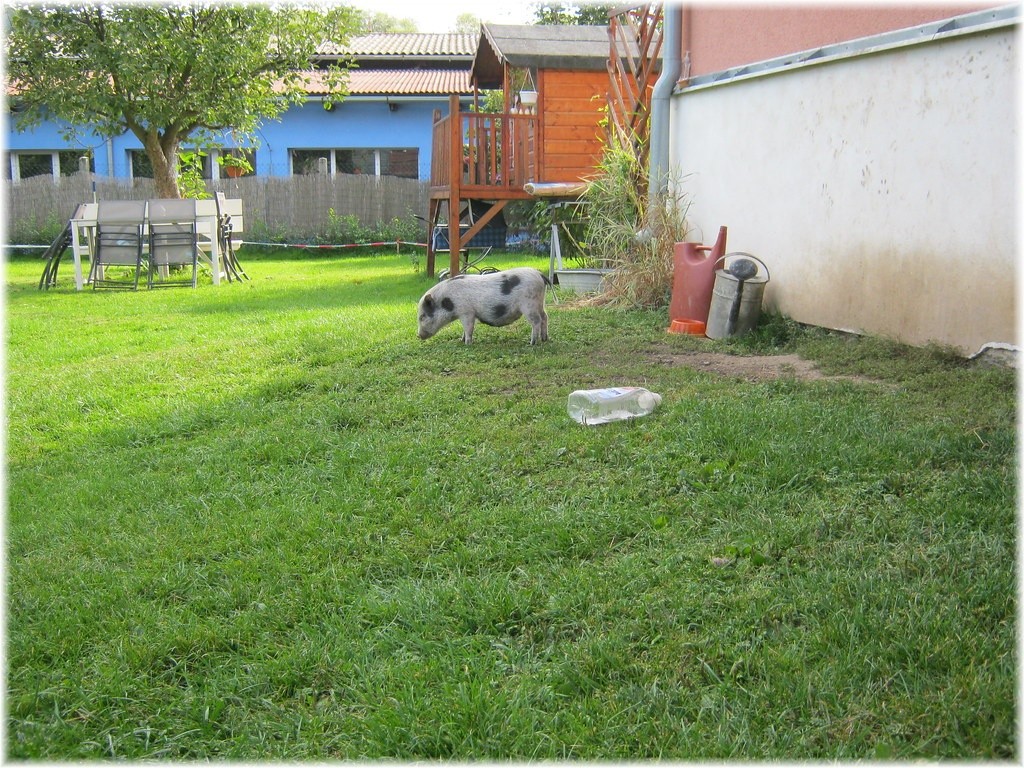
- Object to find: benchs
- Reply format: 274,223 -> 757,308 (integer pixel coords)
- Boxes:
82,198 -> 244,275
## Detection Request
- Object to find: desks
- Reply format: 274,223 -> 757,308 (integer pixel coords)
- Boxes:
69,214 -> 229,286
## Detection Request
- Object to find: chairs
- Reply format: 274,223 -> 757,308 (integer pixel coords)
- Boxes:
86,198 -> 146,292
38,204 -> 86,291
147,198 -> 198,289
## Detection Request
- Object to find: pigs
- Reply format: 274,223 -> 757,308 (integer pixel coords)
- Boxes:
413,267 -> 561,347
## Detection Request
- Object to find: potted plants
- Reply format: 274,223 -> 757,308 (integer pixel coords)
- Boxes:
508,69 -> 539,105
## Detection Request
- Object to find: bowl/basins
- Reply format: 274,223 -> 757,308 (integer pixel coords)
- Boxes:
667,319 -> 706,338
554,268 -> 615,293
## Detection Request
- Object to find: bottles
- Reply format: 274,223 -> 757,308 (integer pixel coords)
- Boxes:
567,387 -> 662,425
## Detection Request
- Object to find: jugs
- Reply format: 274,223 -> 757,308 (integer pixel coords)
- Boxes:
670,225 -> 728,322
705,252 -> 770,344
